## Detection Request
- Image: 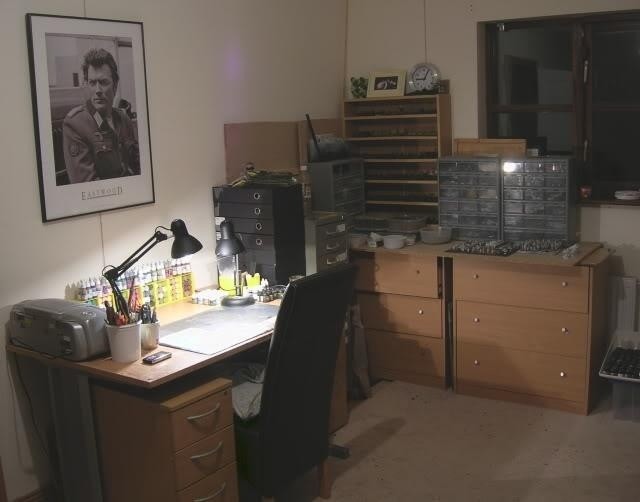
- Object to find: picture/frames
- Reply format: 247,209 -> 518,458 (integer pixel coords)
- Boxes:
25,12 -> 155,222
366,69 -> 406,97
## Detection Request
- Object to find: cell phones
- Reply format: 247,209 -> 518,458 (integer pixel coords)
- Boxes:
142,350 -> 173,363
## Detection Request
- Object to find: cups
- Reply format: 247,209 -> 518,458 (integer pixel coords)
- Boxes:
140,320 -> 161,351
104,319 -> 142,364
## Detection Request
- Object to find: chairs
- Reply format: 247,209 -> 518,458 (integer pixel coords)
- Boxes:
236,263 -> 358,502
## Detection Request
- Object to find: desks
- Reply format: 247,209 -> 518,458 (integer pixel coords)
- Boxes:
6,277 -> 346,502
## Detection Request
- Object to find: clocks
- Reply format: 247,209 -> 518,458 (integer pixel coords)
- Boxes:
408,62 -> 439,92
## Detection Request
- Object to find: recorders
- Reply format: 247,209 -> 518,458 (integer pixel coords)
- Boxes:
8,296 -> 111,362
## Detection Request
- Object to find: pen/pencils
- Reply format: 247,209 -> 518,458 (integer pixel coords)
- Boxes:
105,277 -> 156,326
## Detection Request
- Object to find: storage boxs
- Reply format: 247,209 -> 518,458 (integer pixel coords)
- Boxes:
385,214 -> 428,230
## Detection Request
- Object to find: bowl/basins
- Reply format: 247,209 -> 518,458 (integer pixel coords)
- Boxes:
383,234 -> 407,250
418,227 -> 453,245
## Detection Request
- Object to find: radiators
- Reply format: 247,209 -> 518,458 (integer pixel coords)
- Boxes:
610,275 -> 639,331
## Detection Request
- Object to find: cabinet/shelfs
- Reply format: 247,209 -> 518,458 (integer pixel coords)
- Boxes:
452,250 -> 610,416
327,325 -> 348,435
499,156 -> 577,241
312,157 -> 365,214
343,94 -> 451,228
350,249 -> 450,391
437,156 -> 500,241
223,118 -> 343,214
90,375 -> 240,502
453,138 -> 528,157
302,211 -> 350,275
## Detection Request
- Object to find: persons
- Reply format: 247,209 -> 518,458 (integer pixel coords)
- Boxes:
62,47 -> 140,184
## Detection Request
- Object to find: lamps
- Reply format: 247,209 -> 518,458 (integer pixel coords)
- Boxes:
100,218 -> 202,320
214,216 -> 255,307
